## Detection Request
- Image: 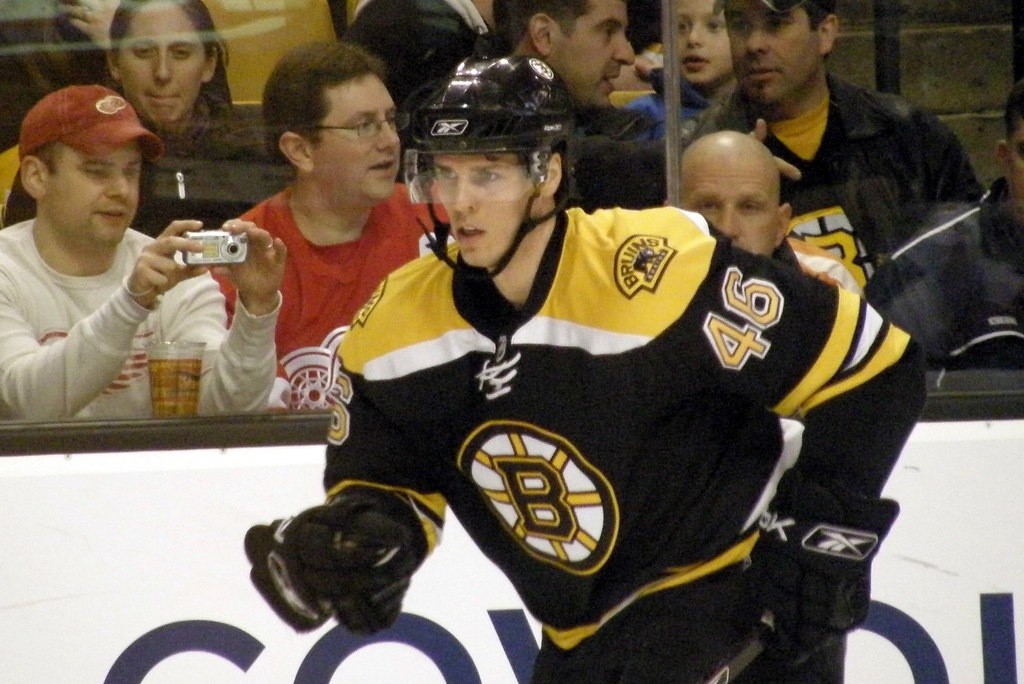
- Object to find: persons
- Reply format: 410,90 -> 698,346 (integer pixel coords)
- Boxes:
245,56 -> 926,684
346,0 -> 1024,372
0,0 -> 452,423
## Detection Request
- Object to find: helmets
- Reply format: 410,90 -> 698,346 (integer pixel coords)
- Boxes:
395,54 -> 577,152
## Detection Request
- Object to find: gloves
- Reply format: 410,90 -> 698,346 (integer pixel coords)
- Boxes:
244,484 -> 428,636
742,467 -> 901,671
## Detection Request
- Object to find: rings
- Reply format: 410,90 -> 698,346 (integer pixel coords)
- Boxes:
81,6 -> 90,20
259,237 -> 275,250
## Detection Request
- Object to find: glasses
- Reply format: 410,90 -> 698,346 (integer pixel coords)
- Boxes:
1008,141 -> 1024,160
309,107 -> 409,139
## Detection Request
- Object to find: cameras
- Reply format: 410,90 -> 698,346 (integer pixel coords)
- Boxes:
181,231 -> 248,265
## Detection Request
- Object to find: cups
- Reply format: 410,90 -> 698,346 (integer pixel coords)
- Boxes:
144,340 -> 206,417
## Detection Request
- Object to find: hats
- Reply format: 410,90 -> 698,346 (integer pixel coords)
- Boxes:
712,0 -> 836,14
18,84 -> 164,162
